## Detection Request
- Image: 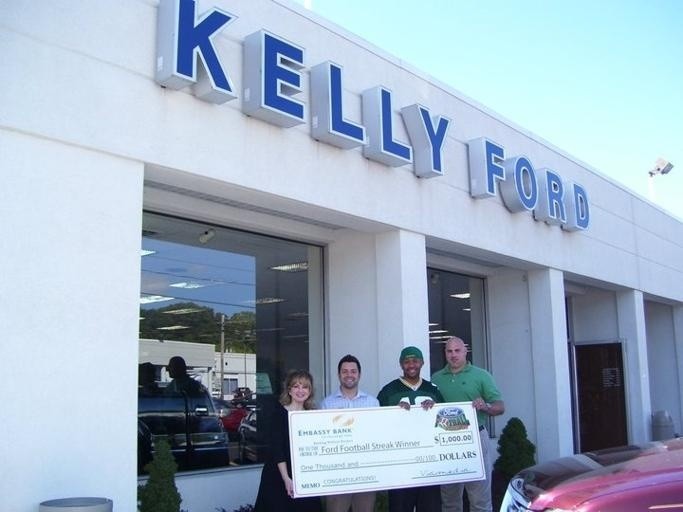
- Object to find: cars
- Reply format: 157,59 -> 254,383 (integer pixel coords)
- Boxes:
213,393 -> 259,465
499,435 -> 683,511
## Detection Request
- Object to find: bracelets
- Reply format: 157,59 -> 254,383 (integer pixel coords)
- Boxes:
486,402 -> 492,410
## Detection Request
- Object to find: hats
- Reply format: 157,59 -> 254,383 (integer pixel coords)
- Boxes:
400,347 -> 423,360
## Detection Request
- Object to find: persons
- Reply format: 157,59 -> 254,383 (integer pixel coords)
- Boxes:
164,356 -> 209,393
252,370 -> 322,512
231,392 -> 248,409
375,346 -> 446,512
139,362 -> 164,396
430,337 -> 505,512
319,355 -> 380,512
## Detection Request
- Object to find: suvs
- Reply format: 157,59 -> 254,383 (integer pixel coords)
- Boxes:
138,381 -> 229,474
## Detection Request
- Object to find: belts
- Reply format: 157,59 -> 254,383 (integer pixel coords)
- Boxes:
479,424 -> 484,431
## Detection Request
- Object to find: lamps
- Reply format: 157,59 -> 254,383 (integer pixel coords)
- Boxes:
649,158 -> 674,202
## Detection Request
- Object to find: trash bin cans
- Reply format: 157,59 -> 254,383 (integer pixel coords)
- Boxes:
652,410 -> 674,442
40,498 -> 113,512
